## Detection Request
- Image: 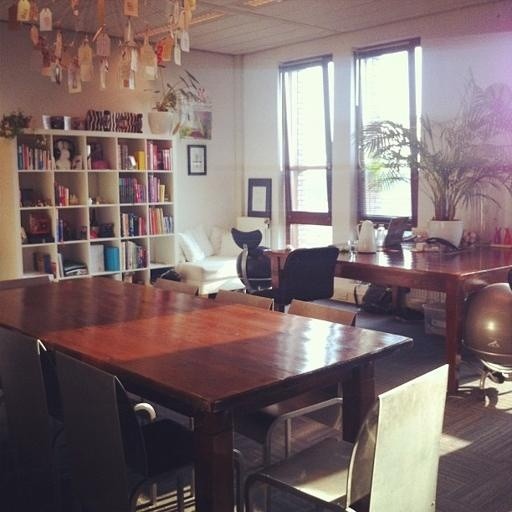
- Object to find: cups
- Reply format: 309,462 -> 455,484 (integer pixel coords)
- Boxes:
336,239 -> 352,252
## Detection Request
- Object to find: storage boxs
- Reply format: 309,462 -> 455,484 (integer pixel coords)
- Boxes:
422,302 -> 449,338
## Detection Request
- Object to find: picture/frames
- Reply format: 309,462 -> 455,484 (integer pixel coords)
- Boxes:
187,144 -> 207,176
248,178 -> 272,218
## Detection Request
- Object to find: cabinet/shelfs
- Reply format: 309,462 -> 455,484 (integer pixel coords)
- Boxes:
0,127 -> 177,282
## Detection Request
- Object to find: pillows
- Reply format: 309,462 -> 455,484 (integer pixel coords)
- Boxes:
179,226 -> 243,262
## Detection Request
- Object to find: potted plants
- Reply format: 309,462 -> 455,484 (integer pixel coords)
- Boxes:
359,67 -> 512,247
0,110 -> 32,139
144,64 -> 199,135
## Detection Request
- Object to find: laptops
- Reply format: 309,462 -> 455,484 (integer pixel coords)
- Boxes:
377,216 -> 408,248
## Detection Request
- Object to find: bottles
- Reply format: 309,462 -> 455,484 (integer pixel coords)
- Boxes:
376,223 -> 387,247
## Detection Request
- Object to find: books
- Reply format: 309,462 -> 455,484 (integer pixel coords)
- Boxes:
17,137 -> 174,284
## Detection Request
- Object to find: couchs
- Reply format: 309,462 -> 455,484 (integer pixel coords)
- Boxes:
176,223 -> 248,299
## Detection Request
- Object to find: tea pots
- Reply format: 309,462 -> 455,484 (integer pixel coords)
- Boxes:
355,219 -> 377,254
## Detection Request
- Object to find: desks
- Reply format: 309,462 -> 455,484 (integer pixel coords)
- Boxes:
263,238 -> 512,391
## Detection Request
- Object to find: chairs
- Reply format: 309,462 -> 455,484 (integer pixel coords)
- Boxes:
231,228 -> 340,311
